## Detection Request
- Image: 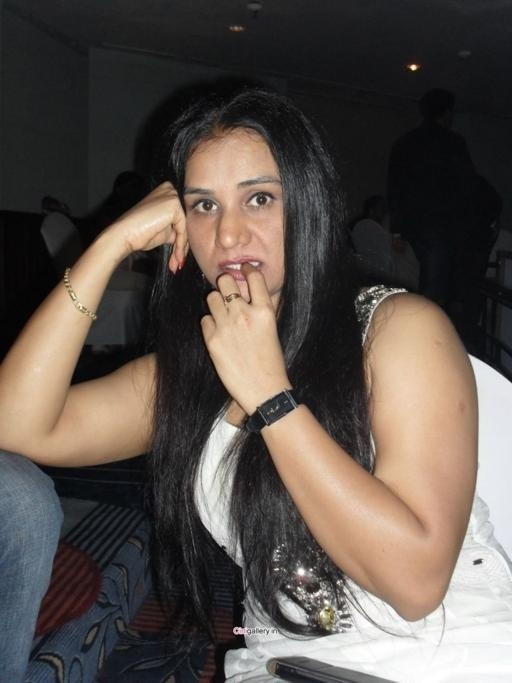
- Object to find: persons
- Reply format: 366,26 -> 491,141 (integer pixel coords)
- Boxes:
1,80 -> 511,683
1,449 -> 64,681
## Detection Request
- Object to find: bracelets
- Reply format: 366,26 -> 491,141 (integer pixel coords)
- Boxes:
62,263 -> 99,322
245,385 -> 306,431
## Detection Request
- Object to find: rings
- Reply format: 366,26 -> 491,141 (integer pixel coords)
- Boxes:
226,292 -> 241,304
